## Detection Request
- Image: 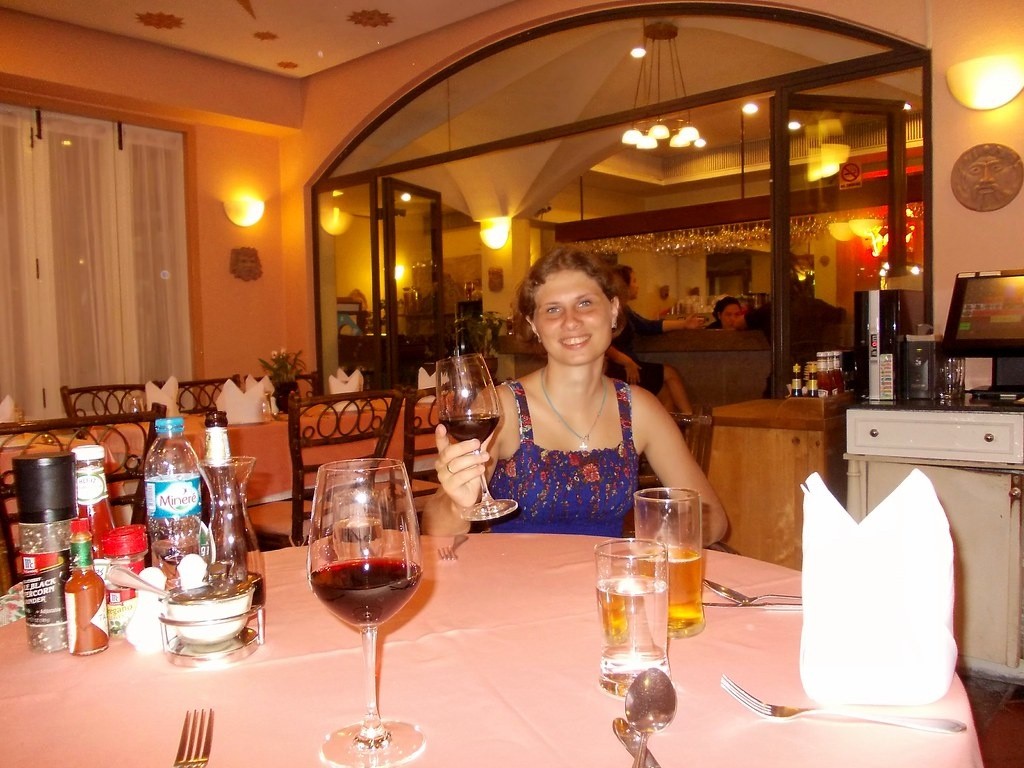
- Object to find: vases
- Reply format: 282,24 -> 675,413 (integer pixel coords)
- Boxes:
274,381 -> 300,414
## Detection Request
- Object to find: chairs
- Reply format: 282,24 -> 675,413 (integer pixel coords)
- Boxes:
0,370 -> 715,595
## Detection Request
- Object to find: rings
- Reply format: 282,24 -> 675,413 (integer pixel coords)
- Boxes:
447,464 -> 456,475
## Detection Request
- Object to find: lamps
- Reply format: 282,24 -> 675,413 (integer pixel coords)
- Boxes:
480,229 -> 508,248
806,119 -> 849,180
826,212 -> 888,252
622,20 -> 699,150
321,209 -> 352,239
944,56 -> 1024,110
223,198 -> 265,227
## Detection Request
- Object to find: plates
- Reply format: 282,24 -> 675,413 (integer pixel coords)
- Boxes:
0,438 -> 27,453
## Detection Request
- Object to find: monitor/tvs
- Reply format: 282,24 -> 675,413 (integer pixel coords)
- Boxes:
943,269 -> 1024,357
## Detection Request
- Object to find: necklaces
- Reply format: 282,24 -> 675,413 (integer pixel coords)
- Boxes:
542,368 -> 607,451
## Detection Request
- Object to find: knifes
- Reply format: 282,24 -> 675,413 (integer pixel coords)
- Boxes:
702,602 -> 802,609
612,718 -> 661,768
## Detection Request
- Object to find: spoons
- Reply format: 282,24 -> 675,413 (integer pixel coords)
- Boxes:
701,578 -> 802,604
624,667 -> 677,768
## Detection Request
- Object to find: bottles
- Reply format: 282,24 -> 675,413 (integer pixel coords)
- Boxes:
17,409 -> 266,656
791,350 -> 844,398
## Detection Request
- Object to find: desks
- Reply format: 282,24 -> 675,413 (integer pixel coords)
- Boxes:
90,395 -> 461,508
0,431 -> 143,543
0,532 -> 985,768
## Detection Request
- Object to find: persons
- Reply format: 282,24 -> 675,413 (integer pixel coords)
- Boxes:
421,243 -> 729,549
603,264 -> 705,415
732,275 -> 847,400
704,296 -> 742,329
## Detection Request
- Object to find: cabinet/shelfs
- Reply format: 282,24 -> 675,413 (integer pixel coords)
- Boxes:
337,332 -> 449,383
711,398 -> 1024,686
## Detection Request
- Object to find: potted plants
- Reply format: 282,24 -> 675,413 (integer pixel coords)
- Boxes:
454,310 -> 506,379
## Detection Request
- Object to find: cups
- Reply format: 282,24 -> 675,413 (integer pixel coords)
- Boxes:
670,292 -> 767,315
125,395 -> 144,413
258,399 -> 272,423
403,262 -> 514,335
633,487 -> 707,640
593,538 -> 670,699
331,474 -> 384,560
935,356 -> 965,400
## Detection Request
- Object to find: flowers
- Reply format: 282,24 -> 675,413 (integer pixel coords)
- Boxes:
258,347 -> 306,386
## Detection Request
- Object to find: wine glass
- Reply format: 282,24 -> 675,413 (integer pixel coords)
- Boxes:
435,351 -> 519,522
306,459 -> 426,767
573,199 -> 923,258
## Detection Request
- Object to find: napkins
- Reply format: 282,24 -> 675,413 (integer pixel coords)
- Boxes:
337,367 -> 364,393
145,375 -> 181,418
1,394 -> 14,422
417,368 -> 449,403
244,373 -> 278,415
799,467 -> 958,707
327,374 -> 362,412
215,377 -> 268,424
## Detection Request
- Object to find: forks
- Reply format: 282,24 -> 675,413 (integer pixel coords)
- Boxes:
436,533 -> 468,562
720,673 -> 967,733
173,708 -> 215,768
